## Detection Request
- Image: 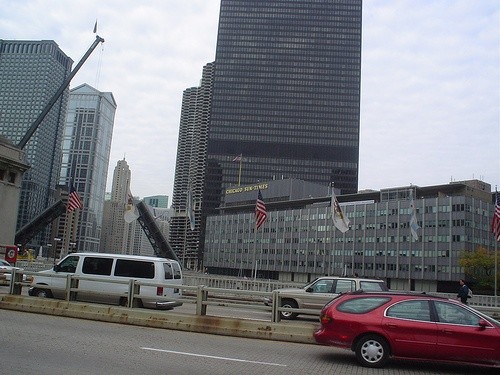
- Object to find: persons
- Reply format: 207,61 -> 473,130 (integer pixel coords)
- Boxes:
458,279 -> 468,304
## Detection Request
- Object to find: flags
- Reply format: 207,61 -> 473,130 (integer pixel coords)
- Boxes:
188,189 -> 195,230
491,191 -> 500,242
331,190 -> 350,233
68,185 -> 82,212
124,185 -> 139,223
256,189 -> 267,229
410,191 -> 421,240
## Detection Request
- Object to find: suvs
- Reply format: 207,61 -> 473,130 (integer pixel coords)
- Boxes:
262,275 -> 388,320
313,288 -> 500,371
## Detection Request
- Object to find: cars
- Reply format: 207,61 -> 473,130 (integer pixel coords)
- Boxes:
0,258 -> 26,287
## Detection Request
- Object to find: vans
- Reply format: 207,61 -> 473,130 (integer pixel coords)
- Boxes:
28,252 -> 185,311
